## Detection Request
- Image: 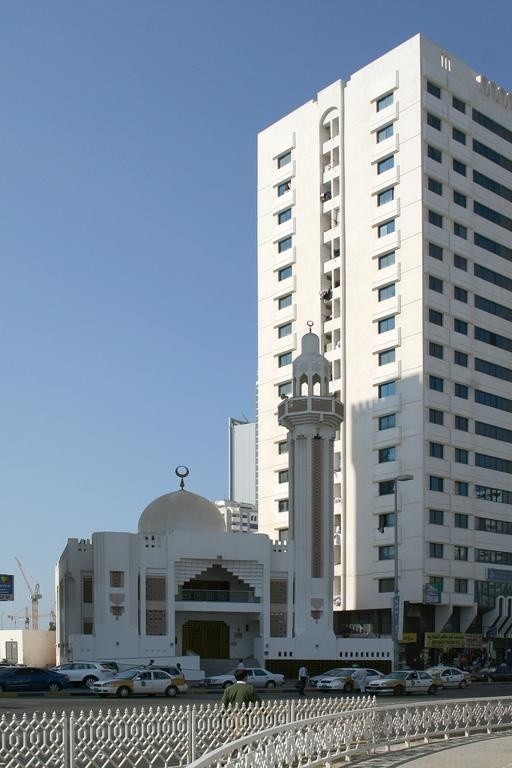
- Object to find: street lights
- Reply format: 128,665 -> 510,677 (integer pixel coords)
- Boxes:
390,471 -> 417,670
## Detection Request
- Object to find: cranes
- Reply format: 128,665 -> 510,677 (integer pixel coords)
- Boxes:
3,551 -> 57,629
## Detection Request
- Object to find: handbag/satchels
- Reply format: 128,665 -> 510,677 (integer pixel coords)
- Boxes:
296,681 -> 305,688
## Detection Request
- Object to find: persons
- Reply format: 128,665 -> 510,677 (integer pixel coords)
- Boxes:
148,659 -> 154,665
351,668 -> 368,693
239,658 -> 245,668
176,663 -> 182,671
299,667 -> 308,695
220,668 -> 261,710
